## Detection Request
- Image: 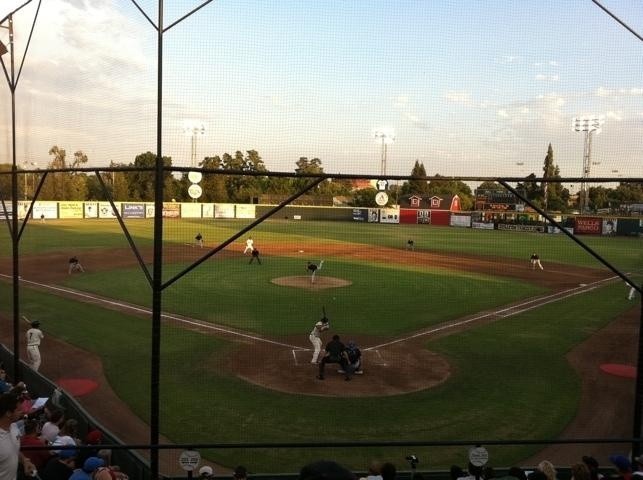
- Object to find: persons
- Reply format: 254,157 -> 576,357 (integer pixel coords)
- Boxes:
248,248 -> 262,265
337,342 -> 364,375
604,221 -> 617,235
192,233 -> 203,249
41,214 -> 44,224
68,256 -> 85,274
25,320 -> 45,371
309,318 -> 330,364
407,239 -> 414,251
317,335 -> 348,380
531,253 -> 543,270
243,236 -> 254,255
304,260 -> 324,284
625,281 -> 637,301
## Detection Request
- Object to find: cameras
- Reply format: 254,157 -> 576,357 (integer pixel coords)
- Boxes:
405,455 -> 418,464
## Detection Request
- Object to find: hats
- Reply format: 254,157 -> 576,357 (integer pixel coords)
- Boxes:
197,464 -> 215,477
69,428 -> 116,480
582,453 -> 643,472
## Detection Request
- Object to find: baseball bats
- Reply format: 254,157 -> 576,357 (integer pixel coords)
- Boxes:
22,315 -> 33,325
322,305 -> 328,323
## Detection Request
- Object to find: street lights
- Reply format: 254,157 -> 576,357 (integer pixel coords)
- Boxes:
370,128 -> 396,176
30,162 -> 37,199
20,159 -> 30,201
182,118 -> 207,168
570,114 -> 603,214
